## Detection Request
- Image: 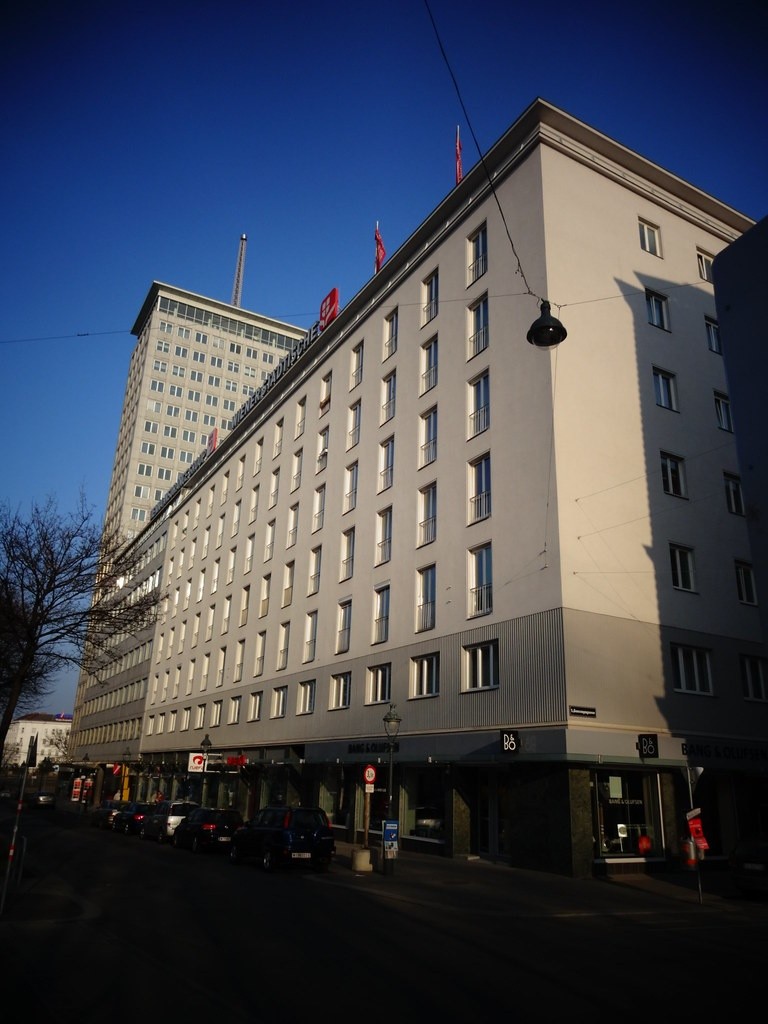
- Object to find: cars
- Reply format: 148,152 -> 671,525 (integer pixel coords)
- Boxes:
731,831 -> 768,901
91,799 -> 131,830
371,791 -> 444,837
30,791 -> 58,808
0,788 -> 13,800
112,800 -> 158,837
171,807 -> 243,857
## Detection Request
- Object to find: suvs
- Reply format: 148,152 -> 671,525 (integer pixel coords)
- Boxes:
137,800 -> 201,844
228,803 -> 337,873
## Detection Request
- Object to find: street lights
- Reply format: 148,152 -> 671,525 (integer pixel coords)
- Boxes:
382,702 -> 401,876
199,733 -> 212,808
76,752 -> 90,811
119,746 -> 132,801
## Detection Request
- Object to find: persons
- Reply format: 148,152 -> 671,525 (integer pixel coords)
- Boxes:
113,790 -> 121,800
151,791 -> 164,804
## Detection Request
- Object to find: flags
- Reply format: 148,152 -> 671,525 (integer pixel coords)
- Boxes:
375,228 -> 386,272
455,129 -> 462,185
